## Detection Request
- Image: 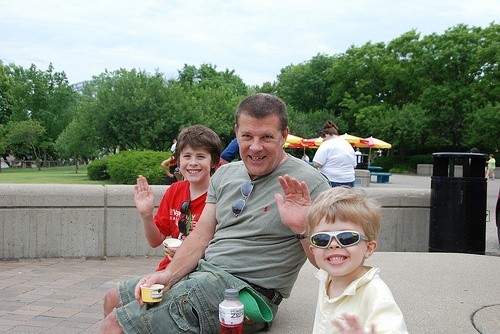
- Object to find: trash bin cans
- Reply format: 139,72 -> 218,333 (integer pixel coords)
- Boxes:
429,152 -> 489,254
356,154 -> 368,170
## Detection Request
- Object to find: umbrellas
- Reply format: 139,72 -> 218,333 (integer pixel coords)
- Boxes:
282,134 -> 392,166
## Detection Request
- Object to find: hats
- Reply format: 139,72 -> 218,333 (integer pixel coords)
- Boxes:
238,288 -> 273,324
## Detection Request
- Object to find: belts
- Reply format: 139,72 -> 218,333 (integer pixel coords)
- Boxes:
243,280 -> 283,305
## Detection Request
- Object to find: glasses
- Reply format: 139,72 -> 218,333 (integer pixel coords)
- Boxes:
178,200 -> 191,234
310,231 -> 368,248
232,181 -> 254,216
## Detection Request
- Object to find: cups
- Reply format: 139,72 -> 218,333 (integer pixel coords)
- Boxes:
140,284 -> 165,303
163,239 -> 182,256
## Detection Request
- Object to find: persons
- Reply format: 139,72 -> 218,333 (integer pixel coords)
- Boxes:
99,93 -> 332,334
355,148 -> 363,163
219,138 -> 242,165
301,153 -> 309,164
307,185 -> 408,334
312,120 -> 357,188
171,139 -> 177,153
161,153 -> 180,185
134,125 -> 221,271
485,154 -> 496,180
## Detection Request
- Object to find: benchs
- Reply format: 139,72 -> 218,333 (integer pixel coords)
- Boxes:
371,173 -> 391,183
368,166 -> 384,172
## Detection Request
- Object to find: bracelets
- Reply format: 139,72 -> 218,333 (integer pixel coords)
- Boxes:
295,231 -> 307,240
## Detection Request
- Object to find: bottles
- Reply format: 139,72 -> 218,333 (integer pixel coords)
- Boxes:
219,289 -> 244,334
